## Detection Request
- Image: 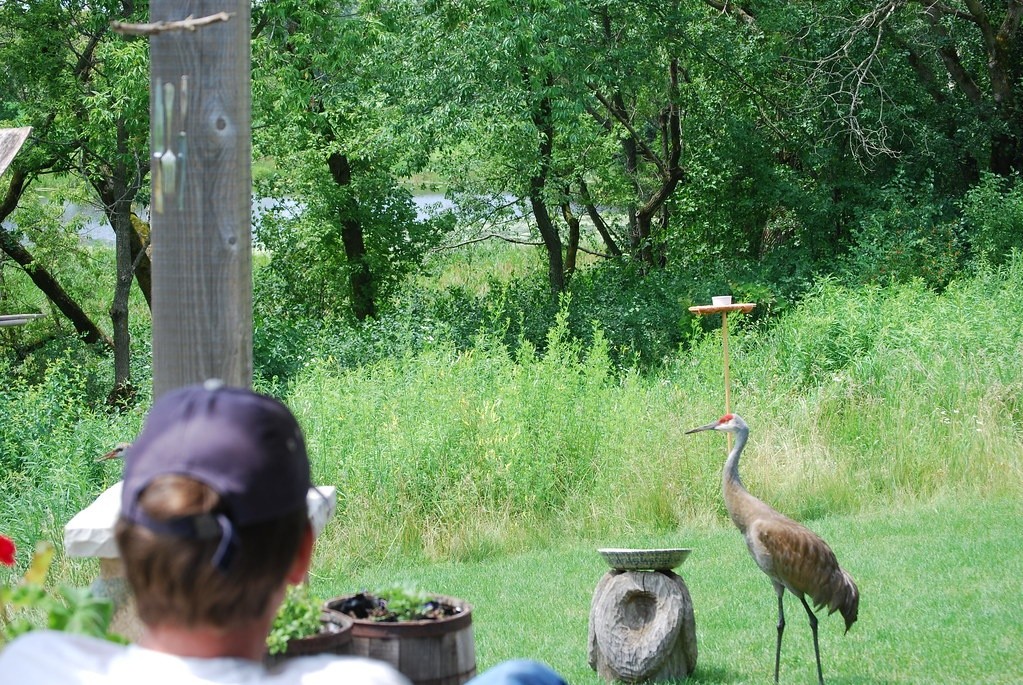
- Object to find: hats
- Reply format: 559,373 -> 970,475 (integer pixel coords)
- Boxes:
121,377 -> 314,572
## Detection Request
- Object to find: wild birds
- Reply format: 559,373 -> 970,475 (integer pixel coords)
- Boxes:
684,413 -> 860,685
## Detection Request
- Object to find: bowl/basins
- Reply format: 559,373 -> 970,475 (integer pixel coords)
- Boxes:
713,296 -> 734,308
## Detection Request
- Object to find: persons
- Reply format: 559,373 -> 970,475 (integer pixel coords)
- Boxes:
1,377 -> 567,685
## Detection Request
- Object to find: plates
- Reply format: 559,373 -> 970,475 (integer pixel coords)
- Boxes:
593,547 -> 695,571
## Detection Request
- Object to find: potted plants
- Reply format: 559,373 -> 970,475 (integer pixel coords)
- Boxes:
321,585 -> 477,685
263,592 -> 354,672
